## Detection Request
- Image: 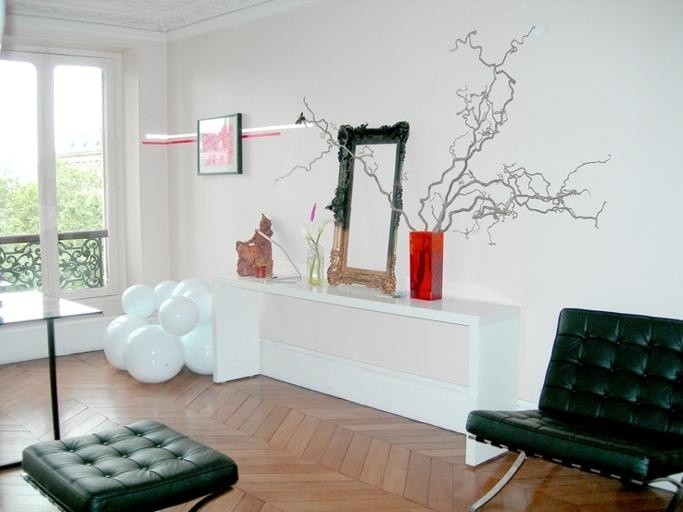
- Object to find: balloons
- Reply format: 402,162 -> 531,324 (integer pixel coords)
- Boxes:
102,277 -> 213,384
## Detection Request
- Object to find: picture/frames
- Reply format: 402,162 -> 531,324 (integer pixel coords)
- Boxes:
197,112 -> 242,175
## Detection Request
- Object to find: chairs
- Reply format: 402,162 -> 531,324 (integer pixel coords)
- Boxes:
466,308 -> 683,512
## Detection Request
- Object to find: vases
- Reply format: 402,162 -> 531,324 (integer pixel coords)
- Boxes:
306,246 -> 325,286
409,232 -> 443,299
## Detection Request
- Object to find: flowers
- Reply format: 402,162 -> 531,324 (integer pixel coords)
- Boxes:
299,203 -> 334,287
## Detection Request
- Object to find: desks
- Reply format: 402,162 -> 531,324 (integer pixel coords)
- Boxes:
212,275 -> 520,468
1,290 -> 102,468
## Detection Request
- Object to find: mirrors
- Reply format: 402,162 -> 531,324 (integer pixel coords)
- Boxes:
324,121 -> 409,295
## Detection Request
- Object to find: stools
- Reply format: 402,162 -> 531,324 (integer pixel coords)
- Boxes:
20,418 -> 238,512
